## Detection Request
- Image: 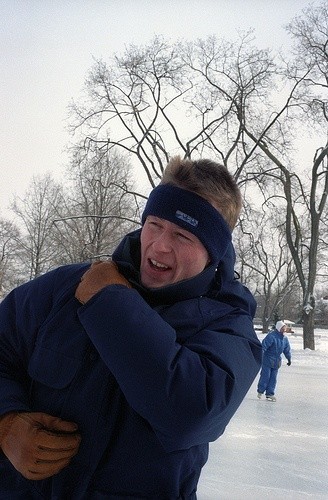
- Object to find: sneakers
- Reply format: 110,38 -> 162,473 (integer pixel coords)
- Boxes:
266,396 -> 276,401
257,391 -> 262,399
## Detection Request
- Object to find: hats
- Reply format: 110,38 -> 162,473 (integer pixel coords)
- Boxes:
276,321 -> 286,331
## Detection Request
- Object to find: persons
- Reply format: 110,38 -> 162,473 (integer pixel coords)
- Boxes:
0,158 -> 264,500
256,321 -> 291,402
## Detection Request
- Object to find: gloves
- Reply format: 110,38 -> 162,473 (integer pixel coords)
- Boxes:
74,257 -> 133,305
1,412 -> 82,481
287,358 -> 291,366
